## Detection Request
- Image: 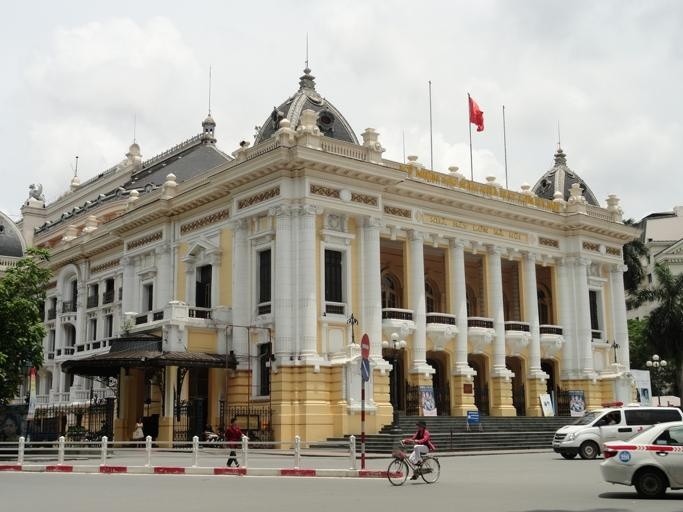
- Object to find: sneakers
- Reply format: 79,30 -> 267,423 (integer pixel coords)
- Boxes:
227,464 -> 239,467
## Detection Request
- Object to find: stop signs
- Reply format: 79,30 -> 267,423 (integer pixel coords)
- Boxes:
360,332 -> 370,360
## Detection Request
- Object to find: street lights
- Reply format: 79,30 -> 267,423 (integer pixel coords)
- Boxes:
380,331 -> 409,435
644,354 -> 667,406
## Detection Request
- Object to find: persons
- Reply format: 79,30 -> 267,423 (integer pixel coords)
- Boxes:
400,419 -> 436,480
133,417 -> 145,447
2,413 -> 22,445
569,394 -> 585,412
643,388 -> 649,405
224,416 -> 250,467
420,391 -> 434,410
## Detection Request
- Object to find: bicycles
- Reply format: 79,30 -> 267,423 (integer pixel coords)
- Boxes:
385,439 -> 441,487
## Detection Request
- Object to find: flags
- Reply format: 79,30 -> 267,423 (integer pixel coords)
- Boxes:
468,96 -> 484,133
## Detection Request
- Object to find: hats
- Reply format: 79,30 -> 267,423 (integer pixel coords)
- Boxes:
416,421 -> 426,428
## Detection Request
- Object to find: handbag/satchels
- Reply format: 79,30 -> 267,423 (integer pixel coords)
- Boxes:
133,432 -> 138,439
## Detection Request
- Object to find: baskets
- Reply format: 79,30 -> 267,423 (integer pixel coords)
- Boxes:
392,448 -> 406,458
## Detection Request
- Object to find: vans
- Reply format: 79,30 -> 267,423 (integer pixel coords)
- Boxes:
549,401 -> 682,462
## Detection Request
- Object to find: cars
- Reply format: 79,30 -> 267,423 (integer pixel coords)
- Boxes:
596,421 -> 682,498
187,422 -> 223,448
